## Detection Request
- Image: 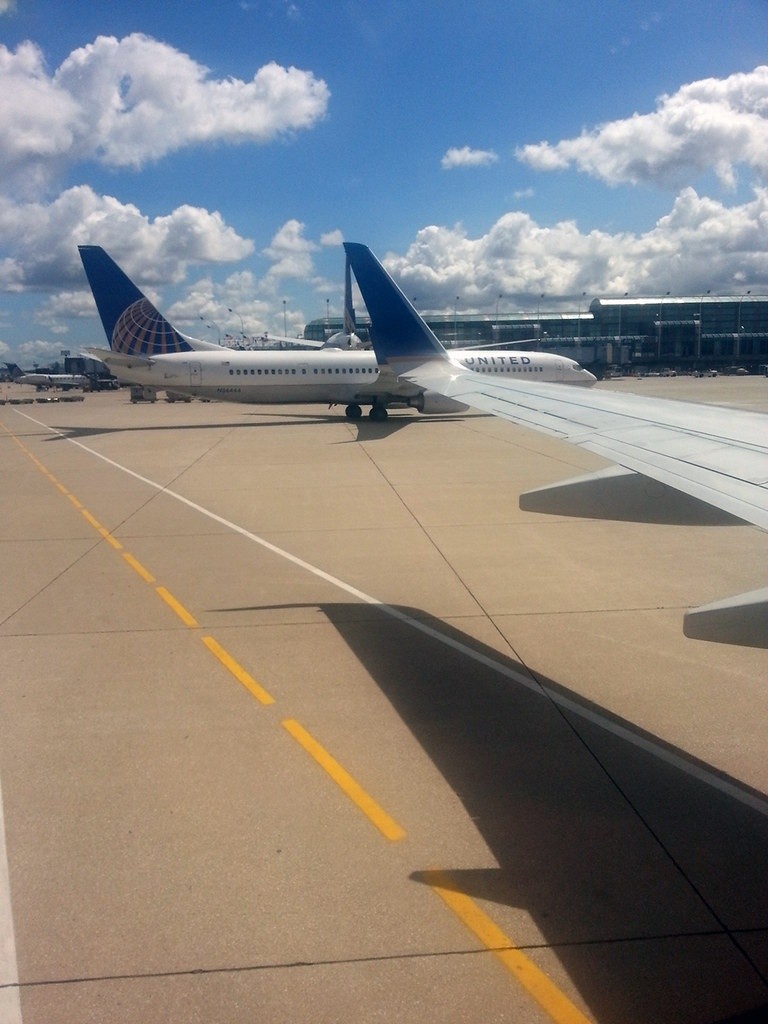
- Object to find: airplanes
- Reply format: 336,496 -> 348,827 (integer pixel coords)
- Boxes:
14,371 -> 93,394
72,242 -> 768,649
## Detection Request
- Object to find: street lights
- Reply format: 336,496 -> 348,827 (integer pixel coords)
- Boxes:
736,291 -> 751,362
577,291 -> 586,354
696,289 -> 711,357
617,293 -> 628,351
658,291 -> 671,358
